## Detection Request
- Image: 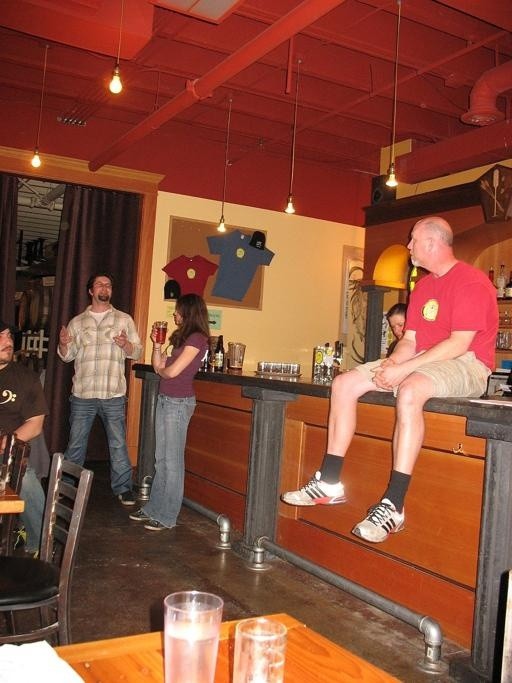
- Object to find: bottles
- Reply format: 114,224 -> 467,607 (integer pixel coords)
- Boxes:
489,265 -> 495,281
505,270 -> 512,300
215,335 -> 224,370
496,265 -> 505,297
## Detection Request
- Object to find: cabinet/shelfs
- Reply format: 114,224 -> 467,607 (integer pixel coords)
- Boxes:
493,297 -> 512,372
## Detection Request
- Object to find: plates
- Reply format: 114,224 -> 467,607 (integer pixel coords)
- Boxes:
254,371 -> 301,375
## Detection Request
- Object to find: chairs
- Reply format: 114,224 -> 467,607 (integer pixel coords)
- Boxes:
1,433 -> 31,635
0,452 -> 95,646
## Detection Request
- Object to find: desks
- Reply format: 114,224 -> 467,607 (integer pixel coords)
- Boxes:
54,612 -> 404,683
0,476 -> 25,513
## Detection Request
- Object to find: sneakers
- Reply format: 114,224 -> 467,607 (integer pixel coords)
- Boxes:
13,526 -> 27,551
129,510 -> 150,521
59,493 -> 64,499
351,497 -> 406,544
32,549 -> 80,570
118,491 -> 136,505
281,470 -> 347,506
144,520 -> 168,531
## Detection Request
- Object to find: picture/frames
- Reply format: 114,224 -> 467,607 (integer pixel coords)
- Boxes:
163,215 -> 267,311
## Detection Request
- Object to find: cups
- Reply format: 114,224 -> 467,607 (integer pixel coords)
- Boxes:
258,361 -> 301,373
154,321 -> 168,343
164,591 -> 224,683
0,462 -> 8,492
232,618 -> 287,683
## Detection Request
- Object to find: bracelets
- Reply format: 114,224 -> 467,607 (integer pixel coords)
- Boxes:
152,346 -> 161,351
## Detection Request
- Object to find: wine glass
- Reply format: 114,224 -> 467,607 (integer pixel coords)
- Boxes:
325,348 -> 333,380
315,345 -> 323,380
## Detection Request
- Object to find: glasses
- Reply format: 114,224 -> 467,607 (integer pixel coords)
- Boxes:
94,282 -> 112,288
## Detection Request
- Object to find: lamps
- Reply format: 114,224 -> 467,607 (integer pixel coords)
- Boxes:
20,237 -> 48,265
284,60 -> 302,214
385,0 -> 401,188
109,1 -> 124,94
31,45 -> 50,168
216,98 -> 233,232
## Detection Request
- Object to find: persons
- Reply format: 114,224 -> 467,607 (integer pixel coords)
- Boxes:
57,272 -> 143,506
0,321 -> 50,560
128,292 -> 210,531
280,215 -> 499,543
385,303 -> 408,356
